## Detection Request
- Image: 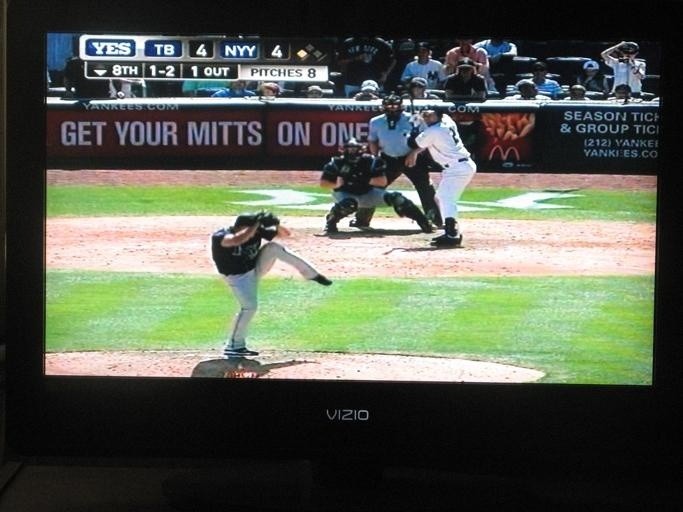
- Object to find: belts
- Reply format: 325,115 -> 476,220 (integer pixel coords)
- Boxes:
445,157 -> 467,167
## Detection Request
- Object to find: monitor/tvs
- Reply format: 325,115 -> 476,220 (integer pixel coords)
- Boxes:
1,0 -> 680,479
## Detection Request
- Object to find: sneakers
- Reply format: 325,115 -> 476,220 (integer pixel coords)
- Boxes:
313,275 -> 330,287
432,233 -> 462,246
323,223 -> 337,232
224,349 -> 258,356
421,222 -> 431,231
349,219 -> 368,225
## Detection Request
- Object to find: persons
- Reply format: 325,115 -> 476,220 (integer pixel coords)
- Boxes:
65,33 -> 648,104
347,94 -> 443,230
311,138 -> 439,238
209,209 -> 329,355
405,104 -> 480,248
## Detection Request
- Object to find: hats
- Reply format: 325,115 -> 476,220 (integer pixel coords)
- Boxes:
583,61 -> 598,70
416,42 -> 429,49
362,80 -> 378,92
533,62 -> 546,69
458,58 -> 475,68
235,214 -> 254,228
411,77 -> 428,87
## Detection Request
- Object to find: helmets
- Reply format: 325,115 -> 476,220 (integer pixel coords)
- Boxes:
421,103 -> 443,119
384,94 -> 402,117
342,137 -> 364,164
